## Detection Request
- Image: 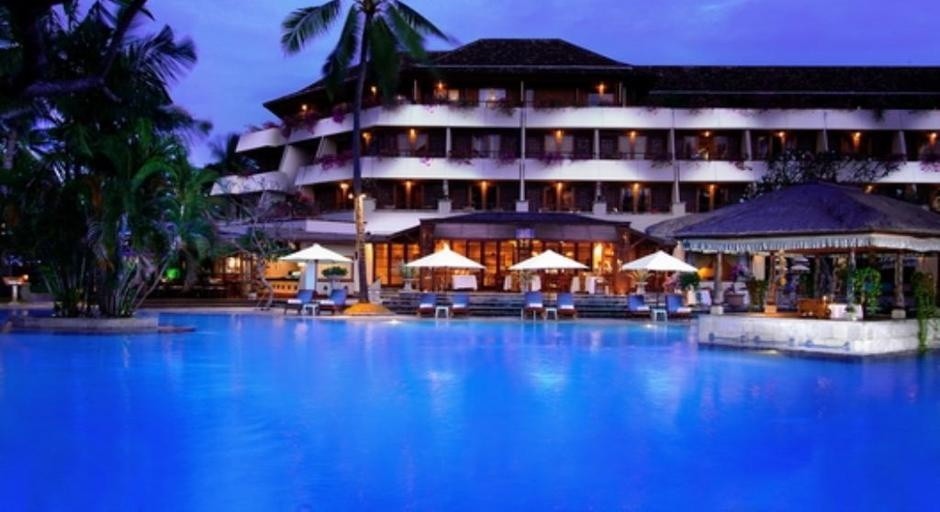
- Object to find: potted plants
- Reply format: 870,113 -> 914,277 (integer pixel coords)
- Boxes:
321,266 -> 347,279
676,271 -> 701,306
626,269 -> 655,294
398,265 -> 417,293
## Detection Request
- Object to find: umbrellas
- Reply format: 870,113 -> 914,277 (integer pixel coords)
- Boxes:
402,243 -> 487,291
278,241 -> 353,302
505,249 -> 590,304
617,247 -> 700,306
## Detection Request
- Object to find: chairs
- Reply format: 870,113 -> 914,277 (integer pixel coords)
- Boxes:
417,291 -> 470,320
519,291 -> 578,322
283,289 -> 347,317
626,294 -> 692,321
